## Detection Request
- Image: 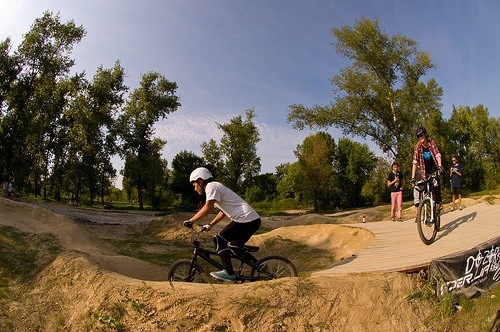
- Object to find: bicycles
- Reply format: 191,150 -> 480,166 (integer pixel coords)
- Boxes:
413,169 -> 443,245
168,222 -> 298,284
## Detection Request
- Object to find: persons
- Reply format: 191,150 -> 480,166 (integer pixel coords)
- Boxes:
183,167 -> 261,283
198,201 -> 203,211
414,186 -> 424,223
450,155 -> 463,211
1,180 -> 12,198
189,203 -> 194,211
387,163 -> 404,223
410,127 -> 443,215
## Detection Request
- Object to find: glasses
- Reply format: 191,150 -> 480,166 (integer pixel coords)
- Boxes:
416,134 -> 425,140
451,160 -> 455,163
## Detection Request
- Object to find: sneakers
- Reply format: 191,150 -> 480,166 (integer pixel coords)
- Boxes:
210,269 -> 236,283
258,265 -> 270,280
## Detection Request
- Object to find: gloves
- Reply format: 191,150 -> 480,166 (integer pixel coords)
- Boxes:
202,224 -> 211,233
183,220 -> 193,229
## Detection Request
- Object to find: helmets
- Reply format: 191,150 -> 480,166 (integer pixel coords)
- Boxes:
415,126 -> 425,136
190,167 -> 213,182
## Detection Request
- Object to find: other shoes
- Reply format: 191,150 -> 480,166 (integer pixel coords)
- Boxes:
415,216 -> 419,224
391,218 -> 395,222
451,208 -> 455,211
459,207 -> 462,210
397,219 -> 403,222
437,204 -> 444,211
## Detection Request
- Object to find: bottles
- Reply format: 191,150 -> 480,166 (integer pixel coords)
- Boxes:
362,214 -> 366,223
